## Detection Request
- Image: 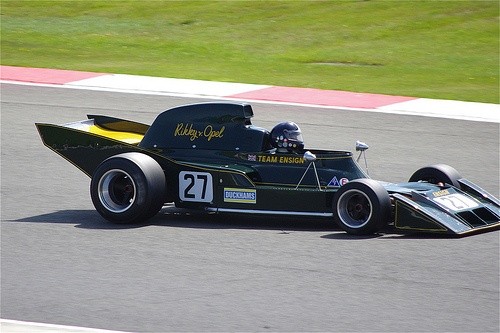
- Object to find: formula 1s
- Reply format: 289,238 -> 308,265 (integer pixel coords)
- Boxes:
34,103 -> 500,239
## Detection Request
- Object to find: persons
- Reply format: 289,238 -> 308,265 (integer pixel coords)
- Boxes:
265,122 -> 305,156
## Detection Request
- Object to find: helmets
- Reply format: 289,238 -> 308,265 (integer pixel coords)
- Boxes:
269,121 -> 305,152
